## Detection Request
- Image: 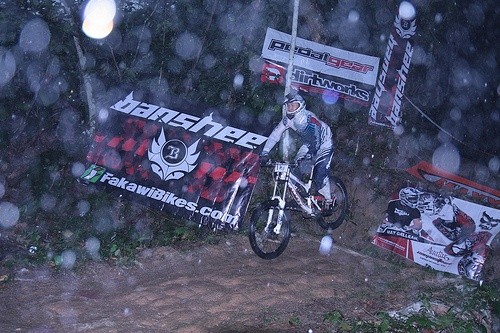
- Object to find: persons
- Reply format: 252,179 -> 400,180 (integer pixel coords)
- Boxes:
260,93 -> 335,205
387,188 -> 421,230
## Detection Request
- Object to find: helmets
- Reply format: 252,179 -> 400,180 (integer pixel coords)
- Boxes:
399,187 -> 421,209
281,90 -> 306,120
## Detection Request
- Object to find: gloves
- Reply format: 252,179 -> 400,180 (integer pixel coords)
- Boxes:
296,156 -> 311,169
259,151 -> 271,167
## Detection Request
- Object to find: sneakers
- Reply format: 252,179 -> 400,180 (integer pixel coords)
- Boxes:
321,195 -> 337,217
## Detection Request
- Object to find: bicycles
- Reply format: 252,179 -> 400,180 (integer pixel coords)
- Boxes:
249,152 -> 348,261
